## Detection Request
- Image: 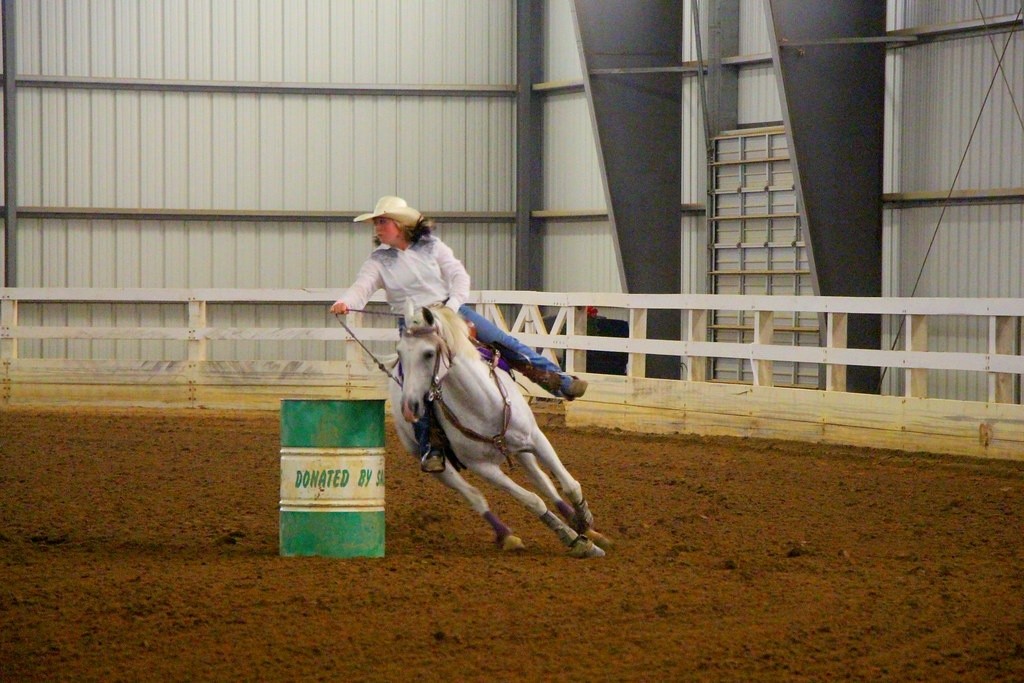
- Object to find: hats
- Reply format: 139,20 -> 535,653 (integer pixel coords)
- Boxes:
353,196 -> 423,231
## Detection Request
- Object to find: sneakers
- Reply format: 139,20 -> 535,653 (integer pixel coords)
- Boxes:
422,454 -> 444,471
564,380 -> 588,401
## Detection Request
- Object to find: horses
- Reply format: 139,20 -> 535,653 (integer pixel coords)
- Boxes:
388,307 -> 617,559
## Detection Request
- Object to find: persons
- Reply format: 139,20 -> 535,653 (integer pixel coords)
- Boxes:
329,196 -> 588,473
